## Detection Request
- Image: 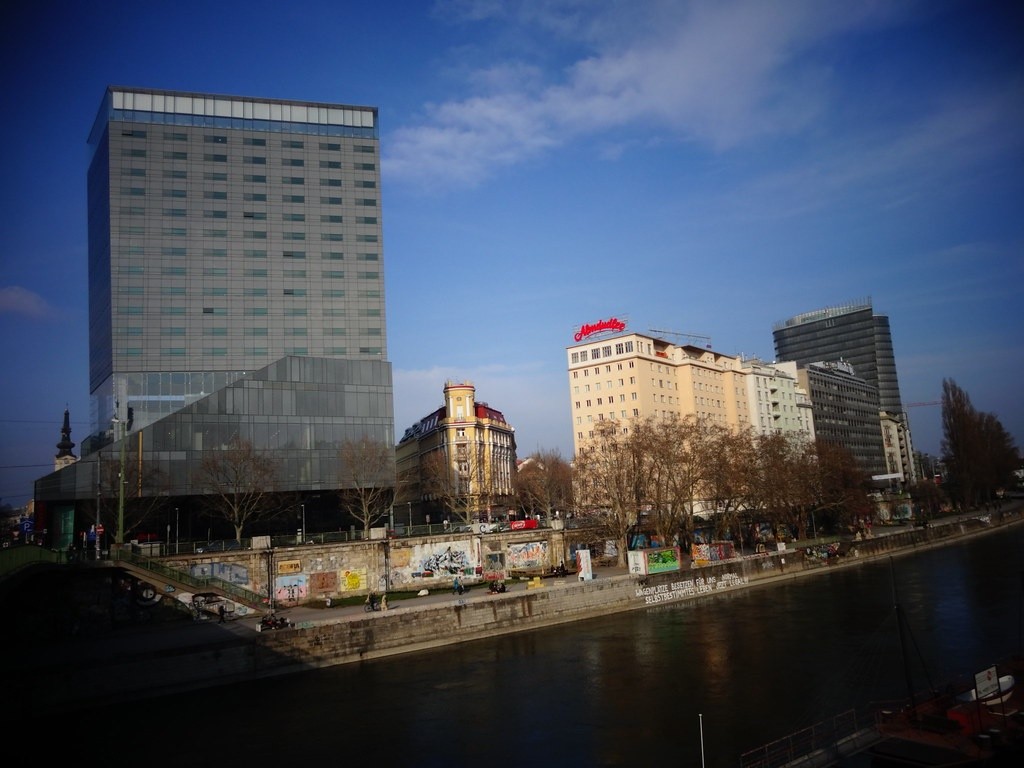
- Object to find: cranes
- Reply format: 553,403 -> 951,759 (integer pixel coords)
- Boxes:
905,400 -> 949,407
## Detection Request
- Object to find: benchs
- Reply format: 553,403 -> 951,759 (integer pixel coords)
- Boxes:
255,618 -> 287,632
800,557 -> 840,570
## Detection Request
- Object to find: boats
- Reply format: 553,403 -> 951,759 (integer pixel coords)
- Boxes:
699,555 -> 1024,768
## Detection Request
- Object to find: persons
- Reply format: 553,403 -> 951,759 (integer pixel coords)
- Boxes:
489,580 -> 505,593
56,543 -> 86,564
218,606 -> 230,624
368,591 -> 388,610
443,520 -> 448,530
551,561 -> 565,578
261,611 -> 286,630
453,576 -> 463,595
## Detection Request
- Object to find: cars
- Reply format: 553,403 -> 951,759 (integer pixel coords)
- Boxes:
194,538 -> 240,554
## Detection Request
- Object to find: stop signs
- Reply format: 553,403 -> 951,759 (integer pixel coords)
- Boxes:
96,524 -> 104,535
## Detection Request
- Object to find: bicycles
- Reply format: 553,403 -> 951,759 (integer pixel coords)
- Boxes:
364,599 -> 381,612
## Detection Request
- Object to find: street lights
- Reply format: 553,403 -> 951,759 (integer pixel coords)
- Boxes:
406,501 -> 412,534
301,505 -> 305,541
175,507 -> 178,554
